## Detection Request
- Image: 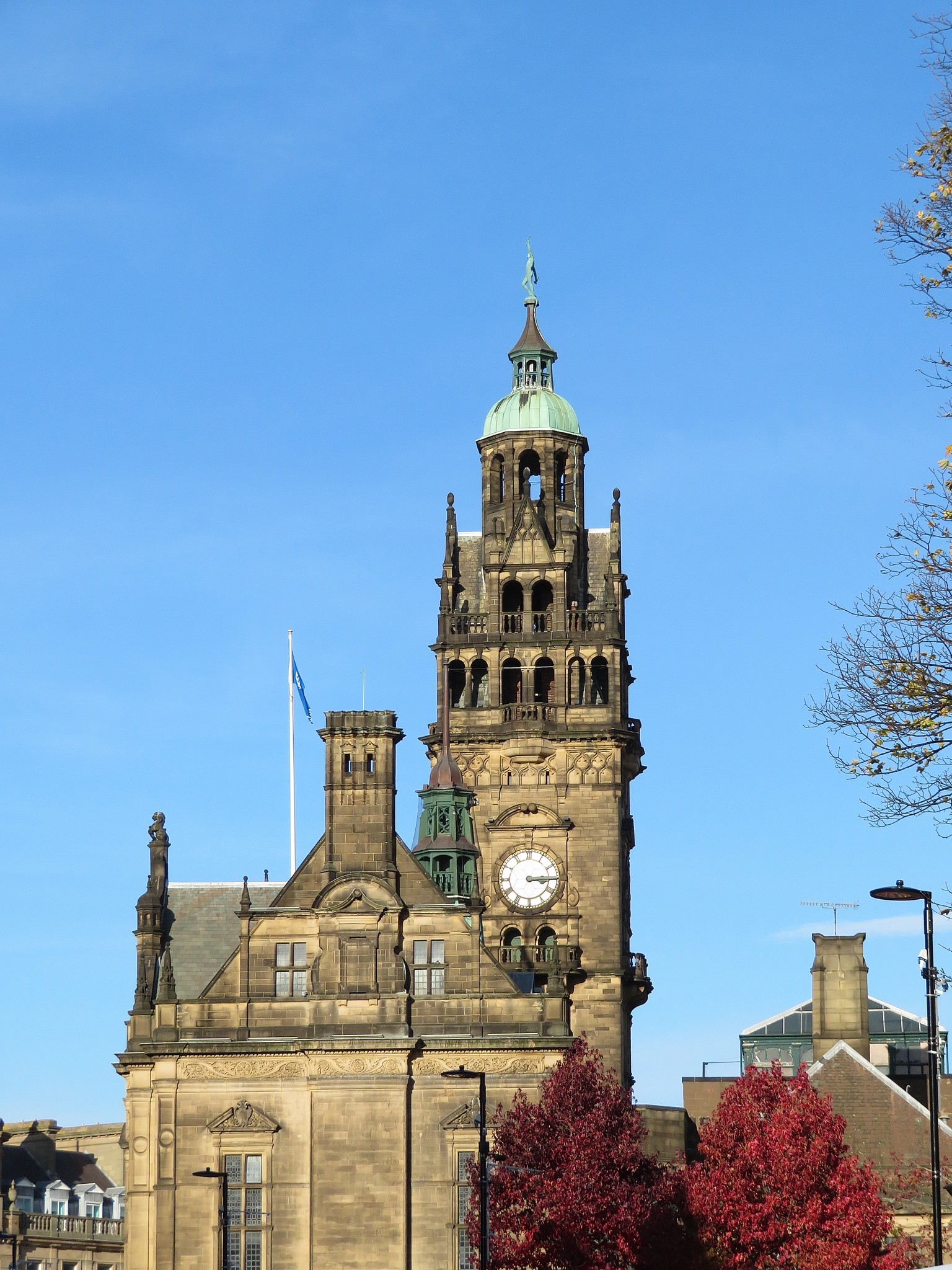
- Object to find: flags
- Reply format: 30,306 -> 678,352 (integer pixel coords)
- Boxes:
291,651 -> 315,724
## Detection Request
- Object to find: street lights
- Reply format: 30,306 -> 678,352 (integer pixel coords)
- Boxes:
871,886 -> 942,1269
441,1066 -> 490,1270
192,1168 -> 232,1270
2,1232 -> 17,1269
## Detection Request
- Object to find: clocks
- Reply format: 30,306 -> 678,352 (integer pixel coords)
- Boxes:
494,843 -> 572,917
